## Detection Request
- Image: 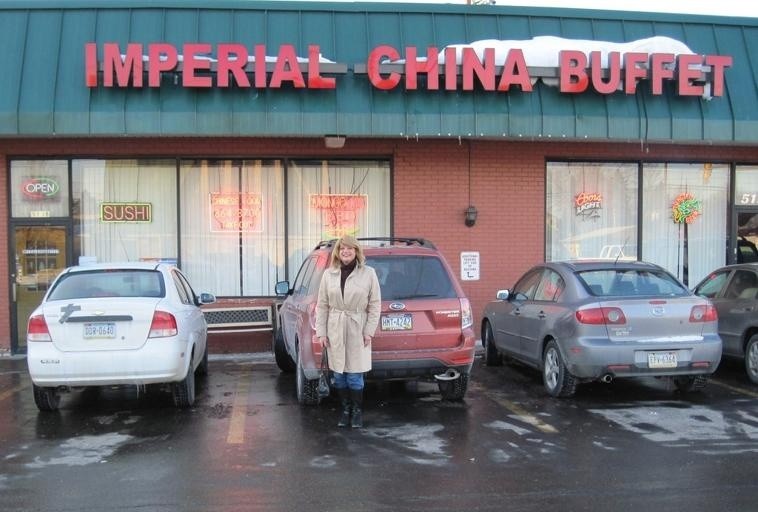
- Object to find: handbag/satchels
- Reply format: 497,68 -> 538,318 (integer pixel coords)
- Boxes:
316,347 -> 332,397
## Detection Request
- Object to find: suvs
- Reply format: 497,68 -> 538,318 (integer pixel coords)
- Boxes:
275,237 -> 476,406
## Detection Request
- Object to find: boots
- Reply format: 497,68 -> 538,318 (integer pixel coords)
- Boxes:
347,387 -> 364,430
334,385 -> 351,429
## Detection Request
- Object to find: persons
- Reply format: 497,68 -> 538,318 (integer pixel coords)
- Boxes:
312,234 -> 382,429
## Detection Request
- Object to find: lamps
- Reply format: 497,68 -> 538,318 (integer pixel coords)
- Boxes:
464,207 -> 478,225
324,134 -> 346,148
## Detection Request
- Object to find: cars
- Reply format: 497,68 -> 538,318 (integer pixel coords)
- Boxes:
600,244 -> 636,258
692,262 -> 758,387
482,261 -> 723,398
27,262 -> 216,411
